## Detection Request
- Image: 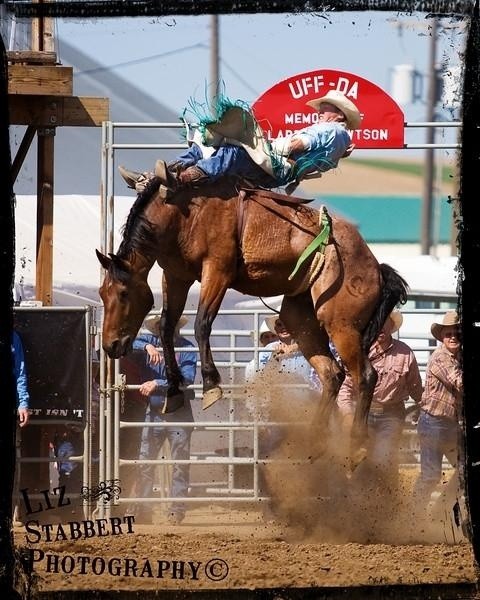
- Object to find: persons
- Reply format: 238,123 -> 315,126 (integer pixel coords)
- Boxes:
132,305 -> 197,524
10,330 -> 30,520
118,88 -> 361,203
53,348 -> 106,494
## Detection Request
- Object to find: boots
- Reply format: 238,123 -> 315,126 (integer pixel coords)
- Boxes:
117,159 -> 210,200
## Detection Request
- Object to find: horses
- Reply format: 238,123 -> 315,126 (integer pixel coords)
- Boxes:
95,171 -> 411,482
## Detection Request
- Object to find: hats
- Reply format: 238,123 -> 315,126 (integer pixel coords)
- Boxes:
145,308 -> 188,335
382,311 -> 403,335
258,306 -> 282,347
305,90 -> 361,132
430,311 -> 462,343
